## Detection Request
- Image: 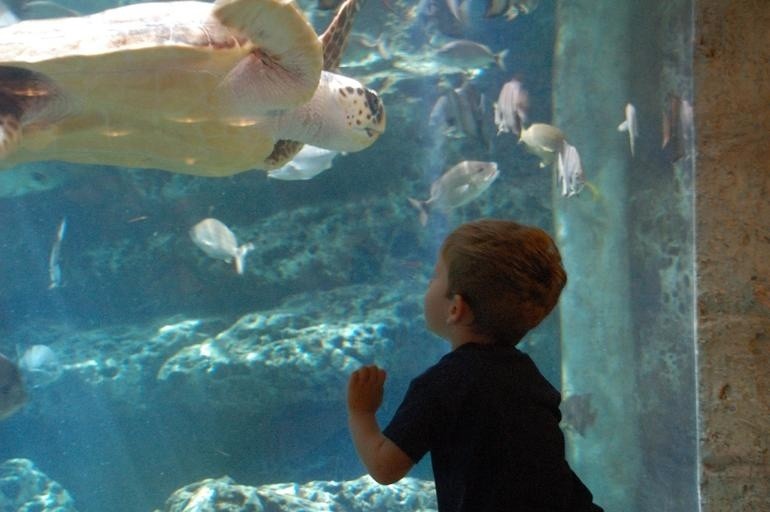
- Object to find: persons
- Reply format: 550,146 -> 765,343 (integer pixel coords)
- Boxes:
348,221 -> 605,511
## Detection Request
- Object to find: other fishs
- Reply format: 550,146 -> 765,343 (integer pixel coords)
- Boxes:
391,0 -> 585,226
